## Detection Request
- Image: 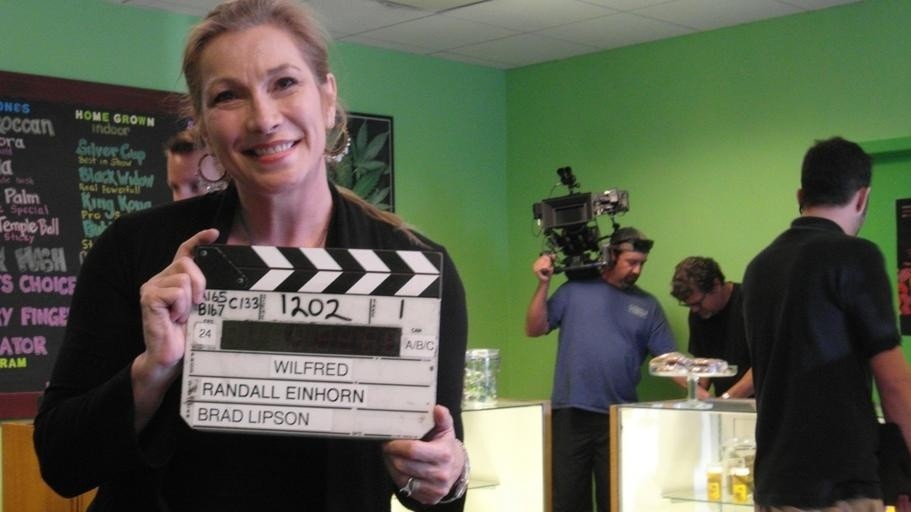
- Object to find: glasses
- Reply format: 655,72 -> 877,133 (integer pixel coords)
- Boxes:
677,290 -> 711,307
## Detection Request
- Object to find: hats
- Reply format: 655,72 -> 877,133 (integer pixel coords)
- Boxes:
608,227 -> 651,248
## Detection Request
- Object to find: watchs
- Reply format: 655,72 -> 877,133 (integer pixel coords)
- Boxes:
438,439 -> 472,507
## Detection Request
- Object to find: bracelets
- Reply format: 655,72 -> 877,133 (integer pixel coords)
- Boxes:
721,392 -> 731,400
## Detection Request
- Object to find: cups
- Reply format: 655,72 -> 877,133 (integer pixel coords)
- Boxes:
705,439 -> 757,500
463,347 -> 503,404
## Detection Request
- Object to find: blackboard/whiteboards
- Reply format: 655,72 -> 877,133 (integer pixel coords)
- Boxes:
0,73 -> 394,416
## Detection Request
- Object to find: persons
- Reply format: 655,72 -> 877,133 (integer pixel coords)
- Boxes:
33,0 -> 472,511
741,136 -> 911,511
164,129 -> 229,202
670,256 -> 754,401
525,227 -> 713,512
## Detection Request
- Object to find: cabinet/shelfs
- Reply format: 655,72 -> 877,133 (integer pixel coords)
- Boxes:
611,400 -> 887,512
391,398 -> 551,512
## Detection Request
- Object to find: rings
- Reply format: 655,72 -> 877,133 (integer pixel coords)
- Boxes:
399,476 -> 420,498
